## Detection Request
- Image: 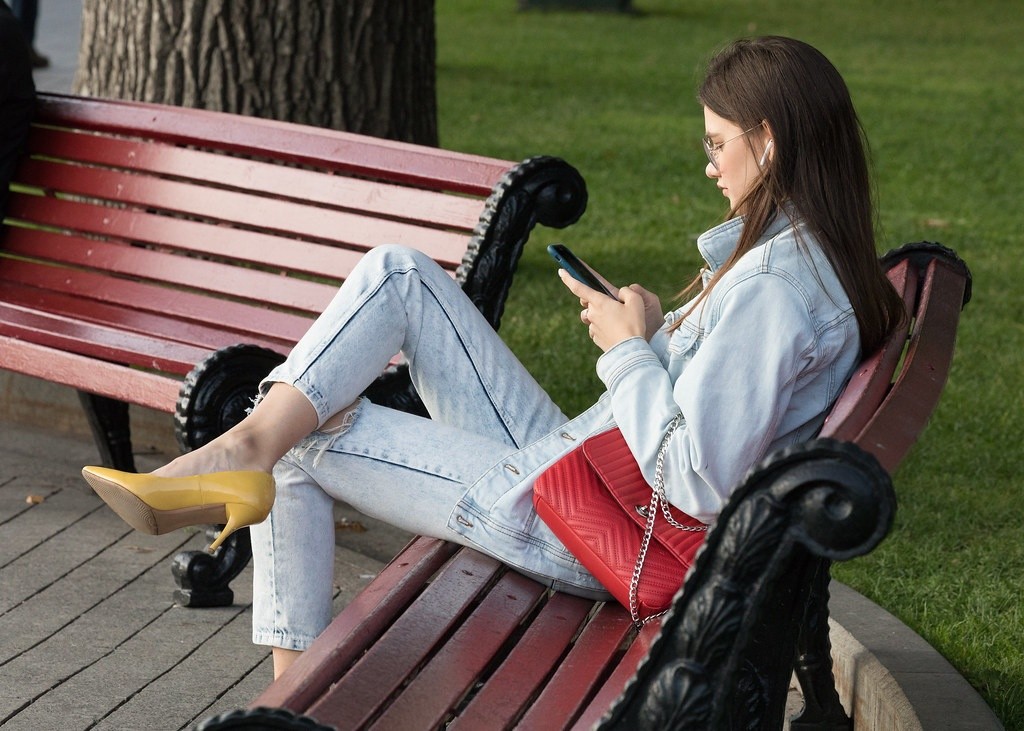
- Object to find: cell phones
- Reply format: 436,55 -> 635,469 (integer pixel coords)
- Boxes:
548,243 -> 618,301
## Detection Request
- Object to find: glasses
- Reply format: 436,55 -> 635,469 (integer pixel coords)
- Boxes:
702,124 -> 762,170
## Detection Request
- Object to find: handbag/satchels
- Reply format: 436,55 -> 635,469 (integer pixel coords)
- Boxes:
532,428 -> 710,619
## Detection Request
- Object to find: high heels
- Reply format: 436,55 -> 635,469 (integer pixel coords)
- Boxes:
82,465 -> 276,554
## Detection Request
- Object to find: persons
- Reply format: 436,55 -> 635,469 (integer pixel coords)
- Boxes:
82,36 -> 904,681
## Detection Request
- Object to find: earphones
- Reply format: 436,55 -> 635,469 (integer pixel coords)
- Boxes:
759,139 -> 773,165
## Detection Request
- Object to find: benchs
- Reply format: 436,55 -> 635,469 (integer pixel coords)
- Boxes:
205,239 -> 974,731
0,87 -> 591,600
